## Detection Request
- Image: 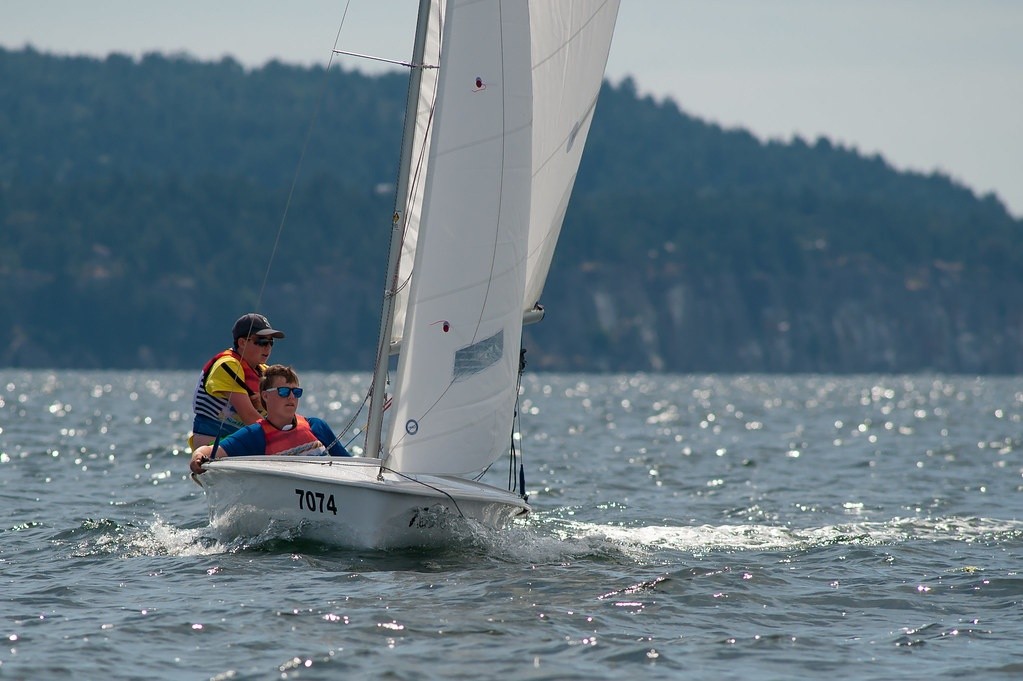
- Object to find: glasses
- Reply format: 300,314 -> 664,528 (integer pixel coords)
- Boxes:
266,387 -> 303,398
245,337 -> 273,346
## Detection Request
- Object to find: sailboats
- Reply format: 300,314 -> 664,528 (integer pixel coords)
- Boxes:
191,0 -> 621,559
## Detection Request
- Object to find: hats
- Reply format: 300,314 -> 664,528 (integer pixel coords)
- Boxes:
233,313 -> 284,340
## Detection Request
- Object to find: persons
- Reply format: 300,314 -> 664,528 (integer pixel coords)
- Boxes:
188,364 -> 351,476
187,314 -> 286,452
